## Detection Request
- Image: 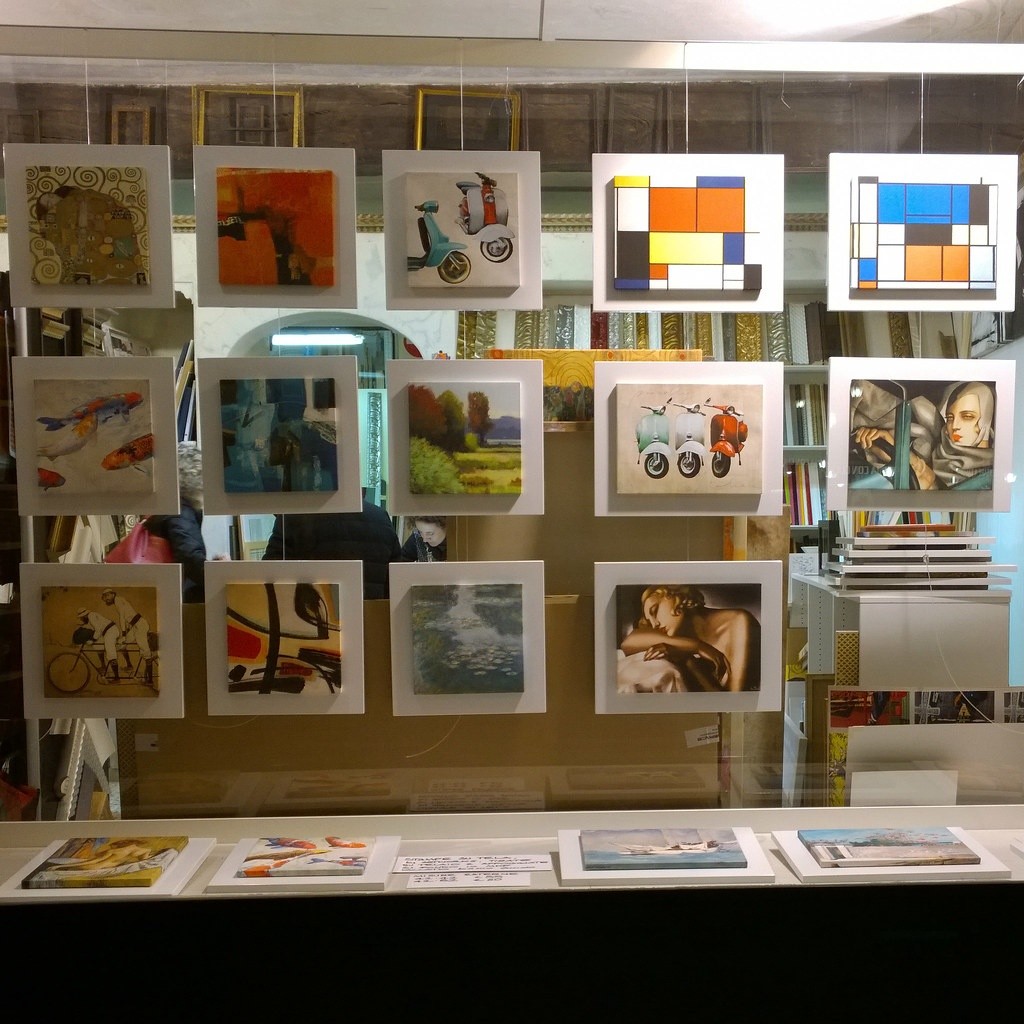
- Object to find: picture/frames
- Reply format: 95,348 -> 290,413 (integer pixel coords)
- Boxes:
0,833 -> 214,898
457,279 -> 784,375
773,828 -> 1013,890
192,144 -> 356,311
591,154 -> 783,314
205,836 -> 398,892
484,348 -> 704,423
556,826 -> 775,893
20,562 -> 185,720
119,598 -> 738,807
828,685 -> 908,804
994,687 -> 1024,724
827,357 -> 1012,515
911,688 -> 990,726
11,355 -> 180,516
0,74 -> 1023,177
829,154 -> 1013,314
2,142 -> 174,308
594,559 -> 774,712
388,561 -> 548,717
967,192 -> 1022,355
196,358 -> 363,513
449,420 -> 734,595
593,363 -> 783,517
202,559 -> 365,716
384,359 -> 545,515
381,149 -> 543,312
103,328 -> 151,355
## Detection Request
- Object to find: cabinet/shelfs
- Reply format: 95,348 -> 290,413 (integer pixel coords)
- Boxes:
781,575 -> 1008,809
789,286 -> 974,679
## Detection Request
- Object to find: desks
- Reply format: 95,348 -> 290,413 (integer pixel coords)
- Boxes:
3,808 -> 1022,903
